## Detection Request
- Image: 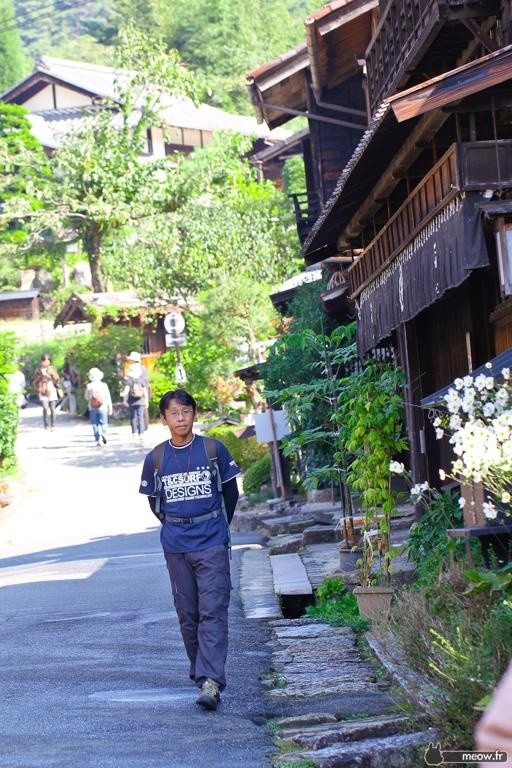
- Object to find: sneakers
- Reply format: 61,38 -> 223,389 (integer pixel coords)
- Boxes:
196,678 -> 221,710
96,434 -> 107,448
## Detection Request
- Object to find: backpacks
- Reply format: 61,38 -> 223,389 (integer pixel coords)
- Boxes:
131,383 -> 143,400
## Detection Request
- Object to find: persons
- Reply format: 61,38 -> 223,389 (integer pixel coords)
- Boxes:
81,367 -> 113,447
127,351 -> 150,430
33,354 -> 64,429
474,664 -> 512,768
122,364 -> 150,442
136,389 -> 244,714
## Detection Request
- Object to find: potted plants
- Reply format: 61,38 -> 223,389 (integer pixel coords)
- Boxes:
329,360 -> 414,636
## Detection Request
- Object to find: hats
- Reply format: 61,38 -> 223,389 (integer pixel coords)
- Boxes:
88,367 -> 104,381
127,352 -> 141,362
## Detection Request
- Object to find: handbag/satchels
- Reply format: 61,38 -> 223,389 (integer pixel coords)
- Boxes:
57,388 -> 63,399
148,386 -> 153,399
90,394 -> 103,408
